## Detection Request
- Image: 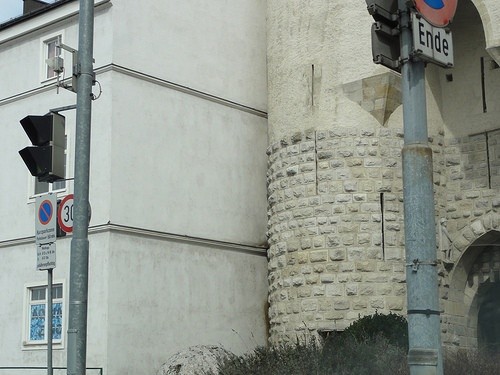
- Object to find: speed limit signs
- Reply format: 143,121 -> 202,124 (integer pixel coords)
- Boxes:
57,194 -> 91,234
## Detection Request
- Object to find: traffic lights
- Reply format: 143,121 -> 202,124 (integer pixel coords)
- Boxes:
19,114 -> 52,178
